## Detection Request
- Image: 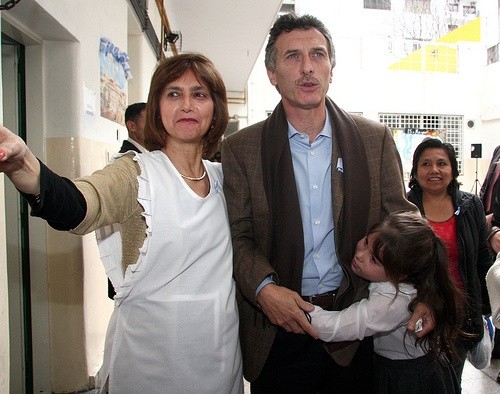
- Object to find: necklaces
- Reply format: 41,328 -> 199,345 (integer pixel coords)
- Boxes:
180,167 -> 206,181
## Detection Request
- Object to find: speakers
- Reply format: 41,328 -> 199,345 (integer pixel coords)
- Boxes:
471,144 -> 481,158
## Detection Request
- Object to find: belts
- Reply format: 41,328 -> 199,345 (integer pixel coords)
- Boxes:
301,289 -> 339,308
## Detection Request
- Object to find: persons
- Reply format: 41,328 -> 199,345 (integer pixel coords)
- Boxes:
406,139 -> 495,386
479,145 -> 500,383
0,52 -> 242,394
300,212 -> 462,394
108,102 -> 227,301
220,14 -> 438,394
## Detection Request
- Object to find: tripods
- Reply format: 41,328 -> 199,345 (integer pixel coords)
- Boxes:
470,158 -> 481,196
